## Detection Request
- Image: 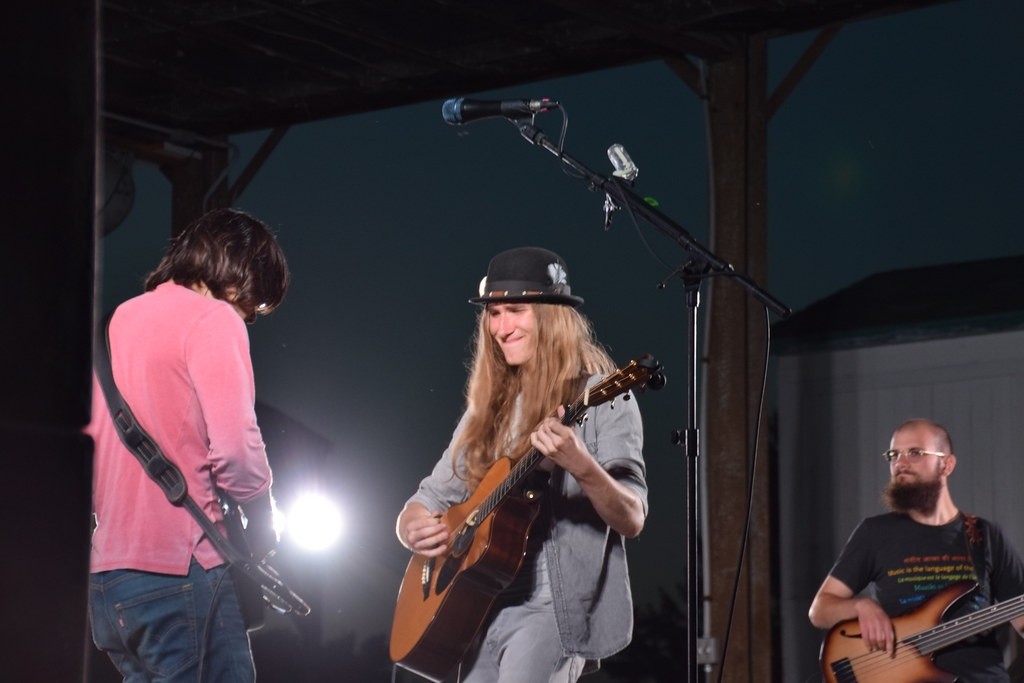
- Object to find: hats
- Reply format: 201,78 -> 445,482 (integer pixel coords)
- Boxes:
469,245 -> 584,306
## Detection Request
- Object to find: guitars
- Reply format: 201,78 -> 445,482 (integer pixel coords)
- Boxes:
387,354 -> 669,683
819,577 -> 1024,682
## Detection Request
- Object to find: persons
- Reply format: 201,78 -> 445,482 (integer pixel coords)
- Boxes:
806,421 -> 1023,683
394,246 -> 650,683
89,209 -> 293,683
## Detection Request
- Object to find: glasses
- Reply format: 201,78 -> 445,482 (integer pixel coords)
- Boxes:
884,447 -> 948,463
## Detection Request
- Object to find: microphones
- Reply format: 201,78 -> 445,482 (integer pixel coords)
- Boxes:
442,97 -> 557,126
608,143 -> 639,185
